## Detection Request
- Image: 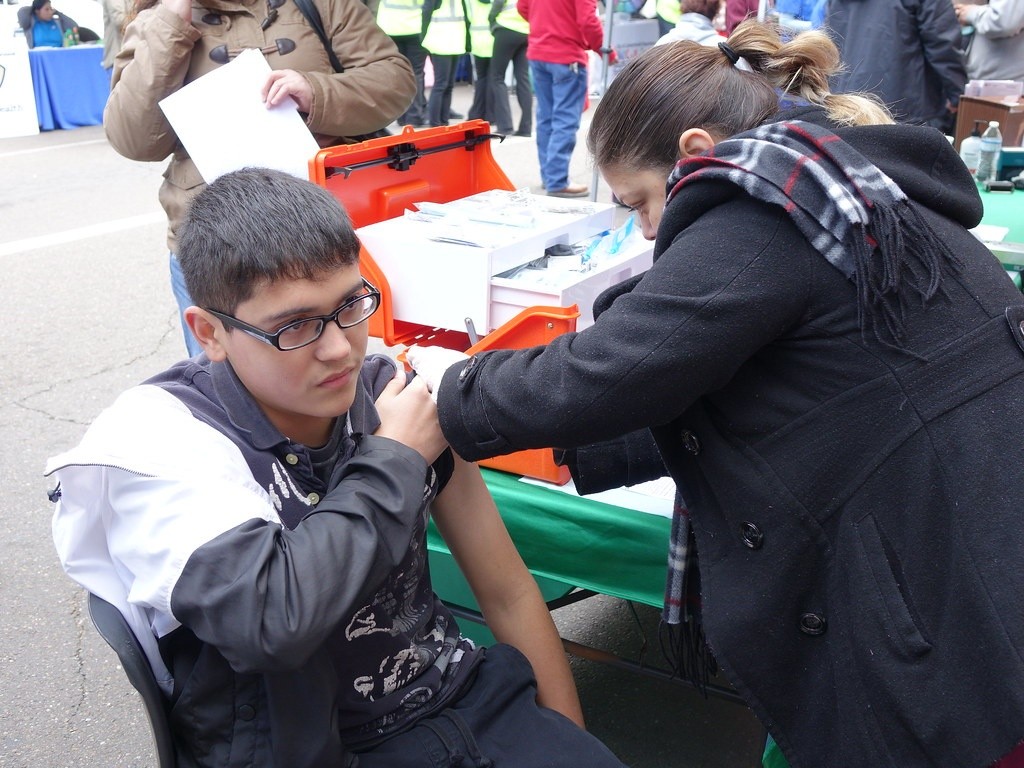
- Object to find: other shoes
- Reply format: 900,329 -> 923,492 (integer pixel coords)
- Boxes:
451,110 -> 464,119
495,128 -> 514,135
514,131 -> 532,138
548,182 -> 589,195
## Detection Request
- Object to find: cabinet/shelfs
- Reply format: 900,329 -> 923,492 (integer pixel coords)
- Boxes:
354,186 -> 654,338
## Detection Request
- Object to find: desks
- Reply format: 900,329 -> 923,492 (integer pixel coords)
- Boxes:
27,39 -> 114,134
425,464 -> 787,768
967,178 -> 1024,297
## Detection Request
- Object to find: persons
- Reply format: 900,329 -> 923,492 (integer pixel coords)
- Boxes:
376,0 -> 533,138
825,0 -> 968,132
619,0 -> 827,71
103,0 -> 417,358
101,0 -> 140,69
17,0 -> 79,47
405,19 -> 1024,768
954,0 -> 1024,83
516,0 -> 618,196
43,167 -> 621,768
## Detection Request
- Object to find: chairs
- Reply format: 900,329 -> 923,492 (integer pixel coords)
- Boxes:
88,590 -> 176,768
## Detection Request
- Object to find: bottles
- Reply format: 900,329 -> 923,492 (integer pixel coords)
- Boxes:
64,28 -> 75,47
73,27 -> 79,45
975,121 -> 1002,192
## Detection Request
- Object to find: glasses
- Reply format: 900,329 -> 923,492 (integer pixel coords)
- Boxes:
205,276 -> 381,349
